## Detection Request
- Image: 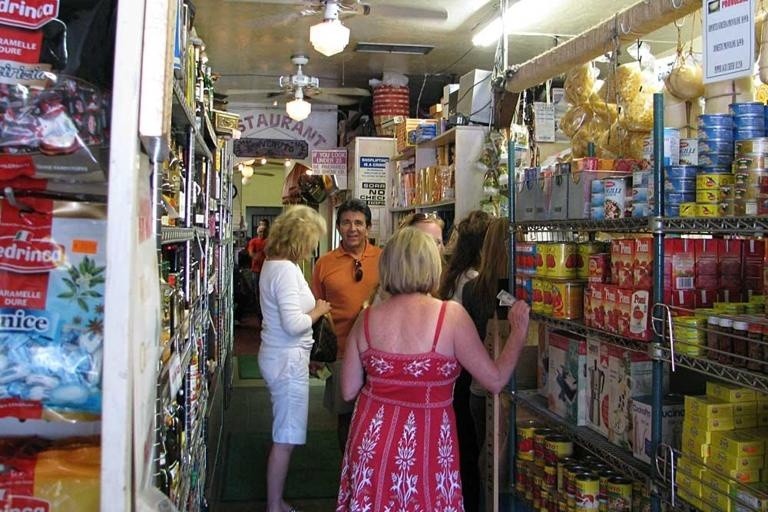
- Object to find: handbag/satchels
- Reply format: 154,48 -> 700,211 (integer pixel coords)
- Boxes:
310,311 -> 338,363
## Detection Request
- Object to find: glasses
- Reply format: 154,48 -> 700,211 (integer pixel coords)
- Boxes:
351,259 -> 362,282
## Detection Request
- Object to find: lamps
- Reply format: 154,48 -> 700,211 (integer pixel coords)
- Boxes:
310,3 -> 350,59
284,88 -> 312,121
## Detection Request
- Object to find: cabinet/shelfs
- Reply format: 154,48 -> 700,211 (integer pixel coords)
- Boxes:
0,2 -> 234,512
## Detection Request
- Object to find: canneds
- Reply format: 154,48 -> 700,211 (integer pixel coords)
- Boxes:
515,239 -> 598,323
663,102 -> 767,218
590,127 -> 698,218
515,418 -> 648,511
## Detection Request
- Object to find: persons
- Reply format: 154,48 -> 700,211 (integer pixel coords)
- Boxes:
259,201 -> 385,512
237,220 -> 270,305
337,211 -> 530,510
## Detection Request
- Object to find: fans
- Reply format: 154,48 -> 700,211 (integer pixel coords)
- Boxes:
241,0 -> 449,32
225,55 -> 371,107
